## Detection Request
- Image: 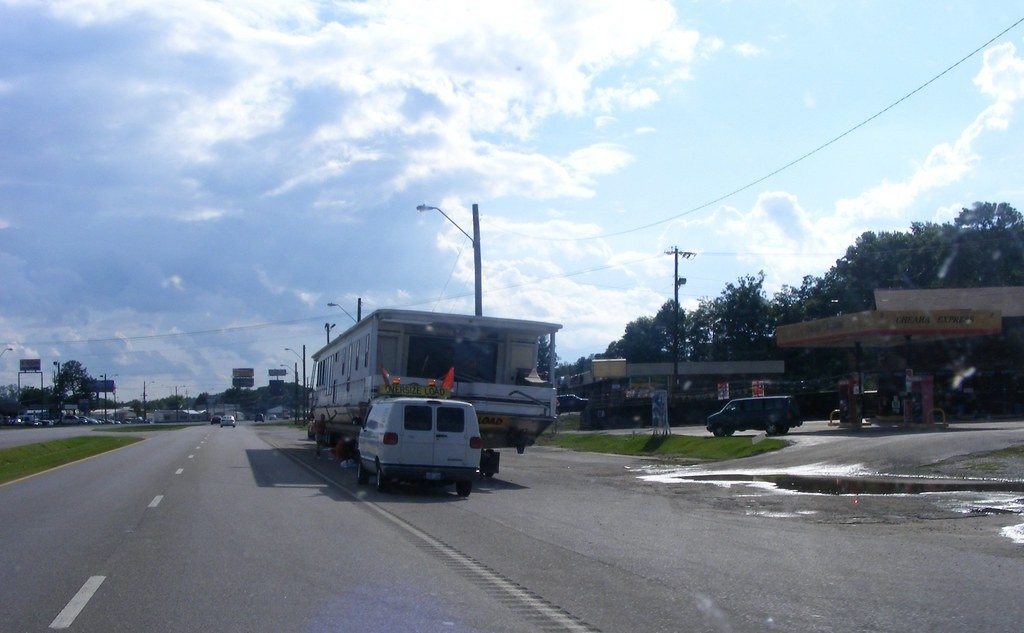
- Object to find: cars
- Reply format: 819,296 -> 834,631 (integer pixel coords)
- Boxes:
220,415 -> 236,428
255,413 -> 264,422
268,414 -> 276,420
0,411 -> 151,427
211,415 -> 222,425
282,412 -> 291,419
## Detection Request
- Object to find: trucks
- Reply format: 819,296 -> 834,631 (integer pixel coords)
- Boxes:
306,309 -> 563,478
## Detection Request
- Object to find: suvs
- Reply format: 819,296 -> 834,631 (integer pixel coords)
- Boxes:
557,395 -> 588,415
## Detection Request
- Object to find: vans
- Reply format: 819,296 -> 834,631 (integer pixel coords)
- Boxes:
706,395 -> 803,437
349,368 -> 483,493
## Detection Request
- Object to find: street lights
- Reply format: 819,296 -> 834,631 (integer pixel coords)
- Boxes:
100,373 -> 107,422
175,385 -> 186,422
280,362 -> 297,425
53,361 -> 61,422
285,345 -> 305,425
144,381 -> 155,421
416,204 -> 482,316
